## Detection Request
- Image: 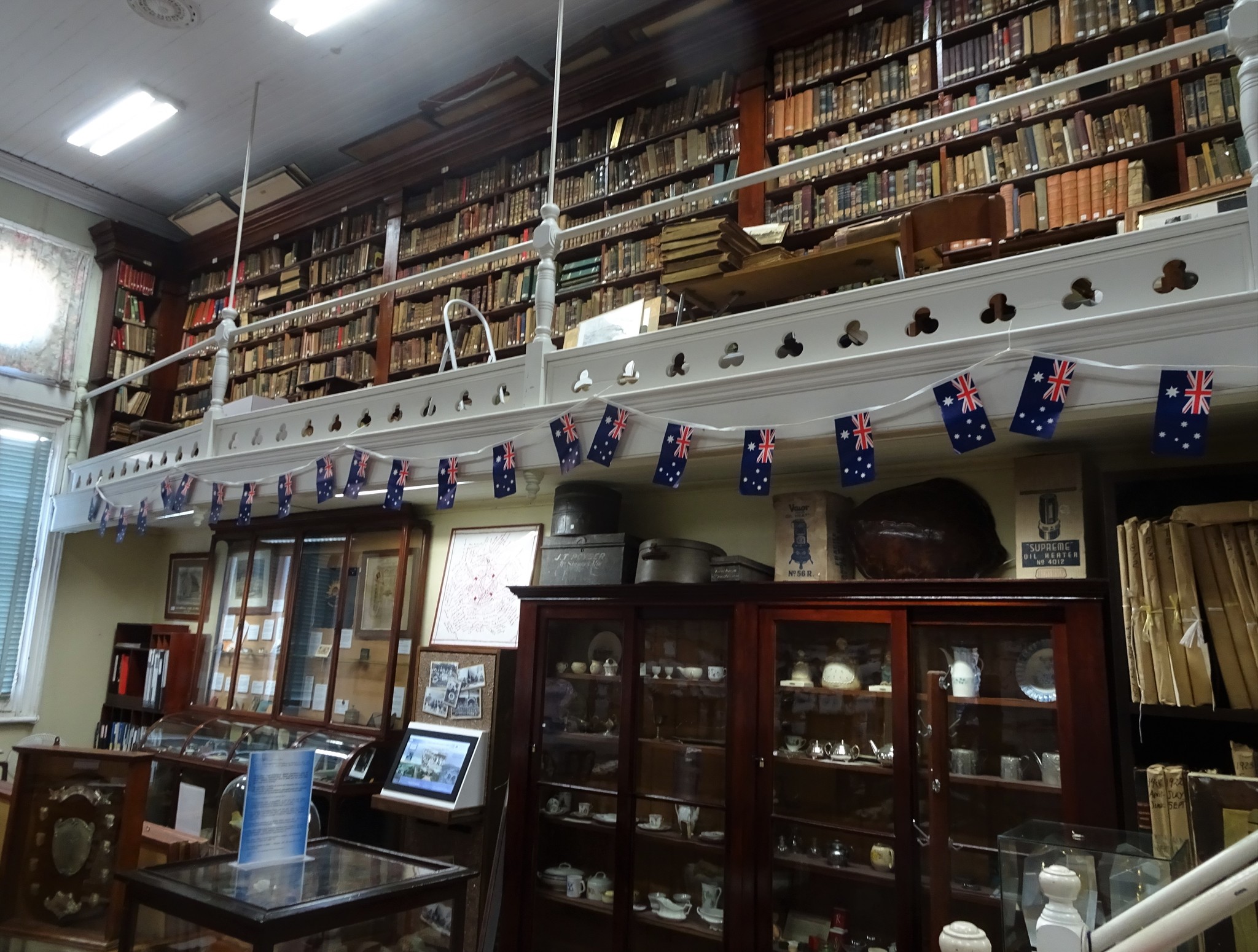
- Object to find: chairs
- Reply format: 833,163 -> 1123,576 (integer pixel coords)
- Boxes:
902,192 -> 1006,279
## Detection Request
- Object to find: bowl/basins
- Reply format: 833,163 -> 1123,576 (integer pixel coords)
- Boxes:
538,863 -> 611,902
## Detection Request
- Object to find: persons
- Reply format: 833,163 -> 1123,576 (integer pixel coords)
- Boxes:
424,665 -> 484,716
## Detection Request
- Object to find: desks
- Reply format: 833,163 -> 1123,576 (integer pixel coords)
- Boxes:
675,232 -> 942,327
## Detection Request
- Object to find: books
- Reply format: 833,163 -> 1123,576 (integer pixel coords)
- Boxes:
107,261 -> 157,443
171,0 -> 1252,428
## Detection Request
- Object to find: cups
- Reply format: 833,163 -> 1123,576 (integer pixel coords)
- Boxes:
949,749 -> 1061,787
567,874 -> 587,897
647,880 -> 723,920
652,666 -> 661,679
665,667 -> 673,679
708,666 -> 727,682
829,906 -> 887,952
555,657 -> 618,676
548,798 -> 662,829
677,666 -> 703,681
784,735 -> 806,752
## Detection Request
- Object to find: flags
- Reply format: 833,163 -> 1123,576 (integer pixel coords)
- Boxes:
437,457 -> 459,510
587,404 -> 630,468
88,487 -> 101,522
1150,370 -> 1215,456
343,450 -> 370,499
209,483 -> 226,524
834,411 -> 876,488
493,441 -> 516,499
171,473 -> 194,513
382,459 -> 410,511
236,483 -> 257,525
739,429 -> 775,497
278,473 -> 292,518
161,475 -> 172,511
652,423 -> 694,489
137,497 -> 148,534
933,372 -> 996,455
1008,356 -> 1076,440
115,508 -> 128,543
98,502 -> 113,538
550,412 -> 581,476
316,454 -> 334,504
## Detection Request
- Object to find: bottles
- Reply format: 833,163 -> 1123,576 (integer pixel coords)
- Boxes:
807,737 -> 860,763
343,704 -> 359,725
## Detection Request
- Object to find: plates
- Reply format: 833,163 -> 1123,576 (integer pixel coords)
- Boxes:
634,905 -> 646,913
541,807 -> 725,842
1016,639 -> 1055,702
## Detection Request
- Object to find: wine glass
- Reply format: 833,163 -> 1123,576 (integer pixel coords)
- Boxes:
653,714 -> 663,741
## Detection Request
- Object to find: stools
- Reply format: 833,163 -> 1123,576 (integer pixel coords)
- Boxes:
128,420 -> 179,445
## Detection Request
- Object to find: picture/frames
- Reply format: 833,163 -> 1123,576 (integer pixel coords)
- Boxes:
355,547 -> 421,639
164,553 -> 214,622
226,542 -> 279,614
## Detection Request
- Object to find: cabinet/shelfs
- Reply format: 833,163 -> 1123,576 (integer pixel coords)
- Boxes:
505,577 -> 1121,952
96,498 -> 431,850
114,835 -> 482,951
85,0 -> 1243,457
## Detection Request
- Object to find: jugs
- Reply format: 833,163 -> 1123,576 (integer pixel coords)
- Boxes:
871,842 -> 895,873
940,647 -> 984,698
869,739 -> 894,766
826,839 -> 855,866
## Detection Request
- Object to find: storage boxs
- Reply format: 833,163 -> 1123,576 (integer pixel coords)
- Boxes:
1013,455 -> 1099,578
773,488 -> 855,581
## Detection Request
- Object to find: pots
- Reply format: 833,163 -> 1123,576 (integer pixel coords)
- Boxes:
633,537 -> 728,585
551,485 -> 622,537
